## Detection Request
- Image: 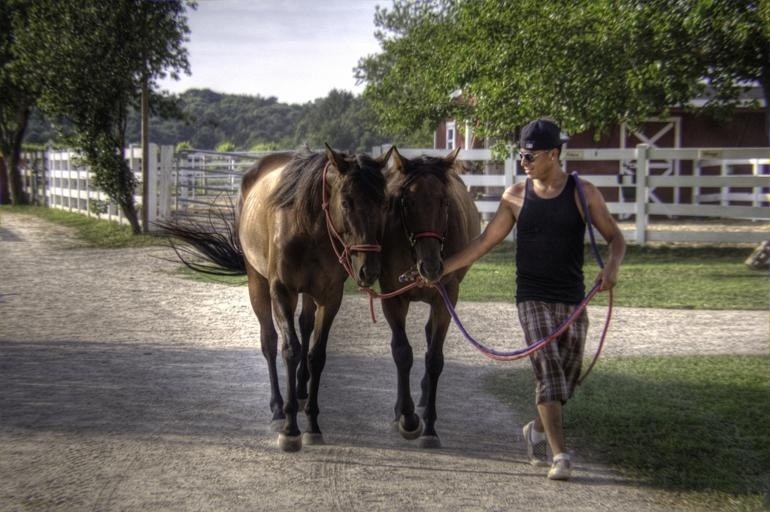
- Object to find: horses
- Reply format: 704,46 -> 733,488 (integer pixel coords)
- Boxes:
145,142 -> 394,453
378,143 -> 481,449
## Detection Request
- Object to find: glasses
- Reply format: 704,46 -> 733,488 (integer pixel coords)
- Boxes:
519,150 -> 548,162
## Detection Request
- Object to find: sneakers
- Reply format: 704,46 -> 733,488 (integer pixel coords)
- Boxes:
522,419 -> 548,467
548,453 -> 572,480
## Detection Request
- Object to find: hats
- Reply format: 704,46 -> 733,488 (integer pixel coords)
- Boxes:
519,118 -> 568,150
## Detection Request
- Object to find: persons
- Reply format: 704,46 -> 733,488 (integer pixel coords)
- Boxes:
419,119 -> 626,479
